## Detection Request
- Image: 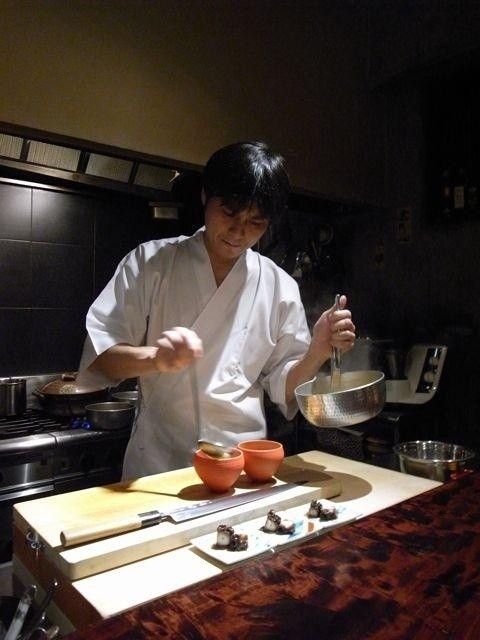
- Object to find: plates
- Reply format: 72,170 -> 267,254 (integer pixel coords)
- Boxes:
189,496 -> 364,567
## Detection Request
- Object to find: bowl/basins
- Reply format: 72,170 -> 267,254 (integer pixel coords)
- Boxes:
238,440 -> 285,484
192,444 -> 245,494
394,438 -> 476,484
110,391 -> 139,404
84,401 -> 137,430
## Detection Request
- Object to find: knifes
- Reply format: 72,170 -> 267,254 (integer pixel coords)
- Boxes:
61,478 -> 310,549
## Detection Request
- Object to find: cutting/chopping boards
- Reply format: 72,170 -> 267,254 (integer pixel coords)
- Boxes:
12,456 -> 343,581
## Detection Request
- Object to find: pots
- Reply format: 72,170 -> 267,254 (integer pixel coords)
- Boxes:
32,371 -> 111,416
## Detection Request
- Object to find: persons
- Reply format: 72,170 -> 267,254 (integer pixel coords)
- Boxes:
74,136 -> 358,479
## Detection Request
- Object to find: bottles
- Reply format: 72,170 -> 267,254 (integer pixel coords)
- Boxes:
439,159 -> 479,224
